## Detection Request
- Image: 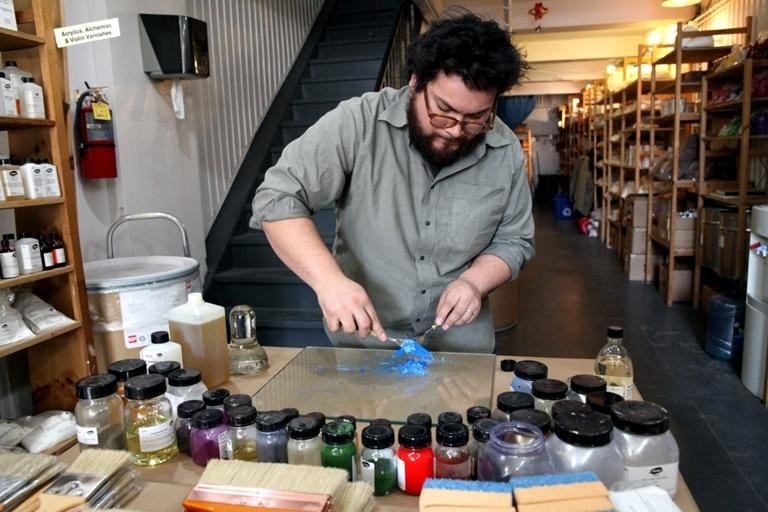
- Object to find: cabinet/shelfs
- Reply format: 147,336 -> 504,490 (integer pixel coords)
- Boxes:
0,1 -> 99,413
510,123 -> 533,184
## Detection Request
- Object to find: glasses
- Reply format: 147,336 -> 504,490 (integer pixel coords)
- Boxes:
423,83 -> 500,135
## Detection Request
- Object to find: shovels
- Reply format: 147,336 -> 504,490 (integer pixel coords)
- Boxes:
339,322 -> 439,359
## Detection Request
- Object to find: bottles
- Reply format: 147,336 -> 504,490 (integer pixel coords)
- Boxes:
75,329 -> 358,482
0,154 -> 61,201
229,304 -> 268,374
0,229 -> 67,280
594,326 -> 634,402
361,356 -> 680,500
0,60 -> 47,120
168,292 -> 231,388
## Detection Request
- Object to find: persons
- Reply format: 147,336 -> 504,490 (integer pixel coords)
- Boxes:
247,14 -> 537,353
576,146 -> 595,216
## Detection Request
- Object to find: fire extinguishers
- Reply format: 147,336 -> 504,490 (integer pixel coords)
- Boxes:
74,82 -> 117,179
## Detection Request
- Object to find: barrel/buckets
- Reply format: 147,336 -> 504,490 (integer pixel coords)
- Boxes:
82,255 -> 201,374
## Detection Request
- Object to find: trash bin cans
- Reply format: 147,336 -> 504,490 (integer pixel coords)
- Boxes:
551,193 -> 574,220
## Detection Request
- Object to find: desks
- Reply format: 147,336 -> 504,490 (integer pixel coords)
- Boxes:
58,342 -> 703,511
